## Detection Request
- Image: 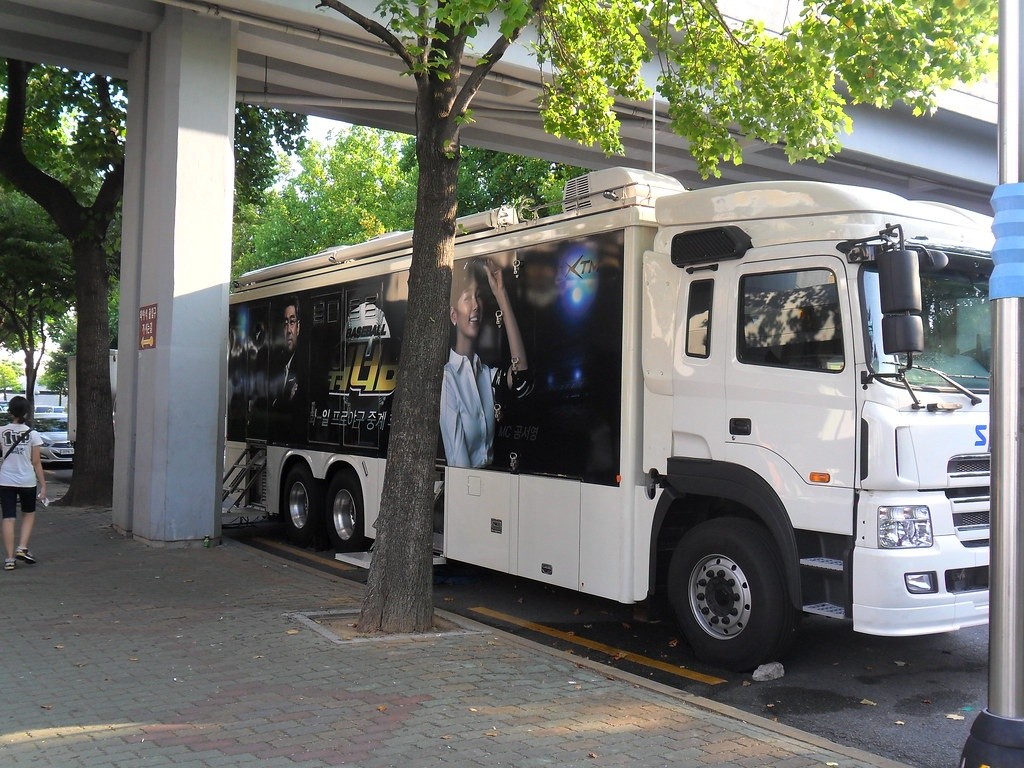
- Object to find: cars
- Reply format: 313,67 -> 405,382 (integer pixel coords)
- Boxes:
32,413 -> 74,467
0,402 -> 68,413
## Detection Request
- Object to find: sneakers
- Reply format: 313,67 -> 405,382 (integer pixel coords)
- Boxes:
15,547 -> 36,564
3,560 -> 16,570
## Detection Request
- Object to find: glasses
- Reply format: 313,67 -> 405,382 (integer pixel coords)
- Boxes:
281,318 -> 298,328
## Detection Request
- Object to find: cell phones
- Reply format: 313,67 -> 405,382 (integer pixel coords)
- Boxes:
38,493 -> 49,507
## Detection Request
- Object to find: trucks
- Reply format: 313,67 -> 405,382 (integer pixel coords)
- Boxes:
66,346 -> 120,452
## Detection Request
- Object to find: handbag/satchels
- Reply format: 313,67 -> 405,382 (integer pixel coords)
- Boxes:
0,457 -> 4,467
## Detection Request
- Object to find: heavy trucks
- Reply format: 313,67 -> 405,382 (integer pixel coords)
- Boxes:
223,167 -> 995,675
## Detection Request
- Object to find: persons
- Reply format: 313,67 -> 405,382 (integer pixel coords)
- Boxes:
0,396 -> 47,570
438,257 -> 534,469
247,301 -> 307,444
